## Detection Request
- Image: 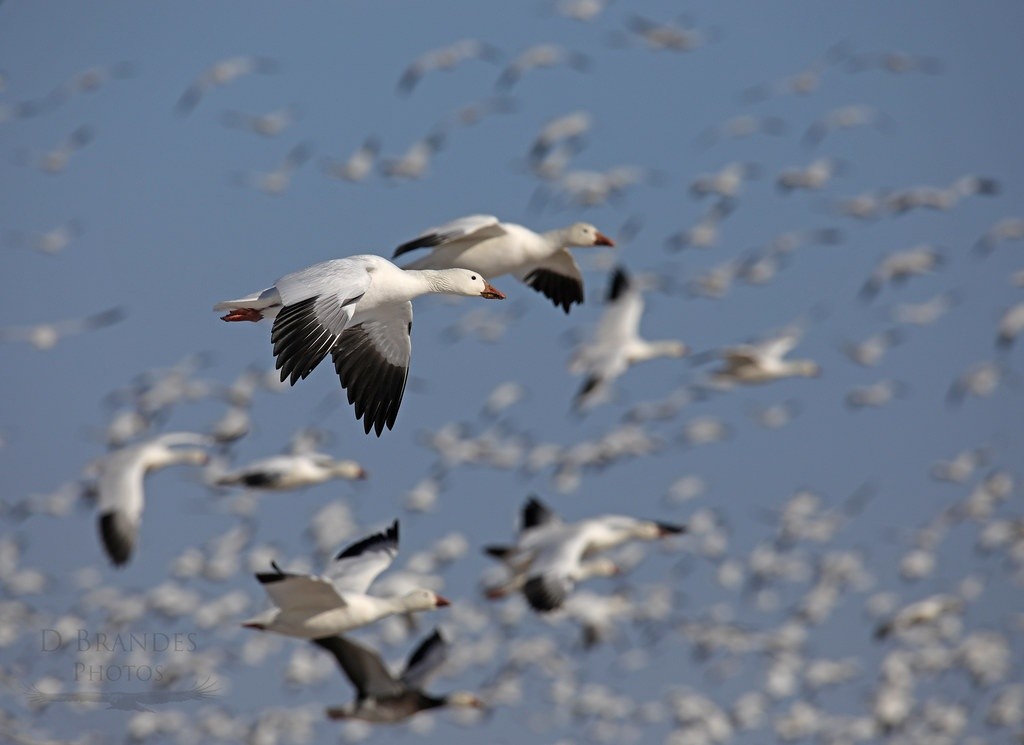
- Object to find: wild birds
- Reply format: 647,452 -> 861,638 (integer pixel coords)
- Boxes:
0,0 -> 1024,745
